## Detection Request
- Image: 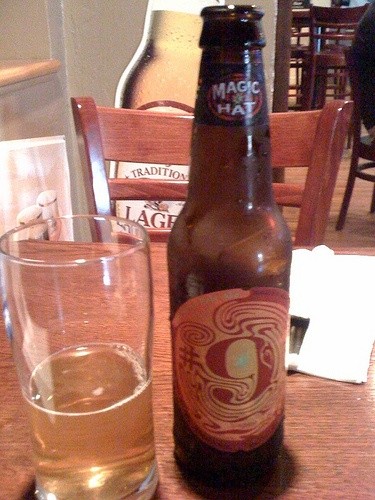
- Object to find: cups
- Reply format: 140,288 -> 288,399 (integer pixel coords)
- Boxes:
1,212 -> 161,500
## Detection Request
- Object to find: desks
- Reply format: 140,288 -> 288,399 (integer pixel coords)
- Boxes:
0,240 -> 375,499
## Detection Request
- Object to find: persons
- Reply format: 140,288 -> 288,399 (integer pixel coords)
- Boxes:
347,1 -> 375,143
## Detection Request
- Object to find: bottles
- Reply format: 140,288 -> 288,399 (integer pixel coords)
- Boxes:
166,4 -> 293,491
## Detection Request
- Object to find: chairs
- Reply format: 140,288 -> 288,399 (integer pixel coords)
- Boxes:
71,2 -> 375,246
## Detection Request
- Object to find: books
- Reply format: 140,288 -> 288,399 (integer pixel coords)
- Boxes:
267,243 -> 375,387
